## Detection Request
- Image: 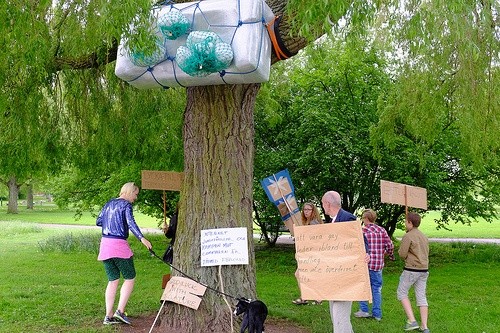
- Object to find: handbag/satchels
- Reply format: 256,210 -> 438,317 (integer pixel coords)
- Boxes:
160,243 -> 174,263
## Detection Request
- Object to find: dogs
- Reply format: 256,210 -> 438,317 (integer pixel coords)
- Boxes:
232,296 -> 269,333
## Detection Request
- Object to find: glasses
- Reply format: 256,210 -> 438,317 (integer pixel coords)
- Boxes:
303,208 -> 313,212
132,182 -> 136,187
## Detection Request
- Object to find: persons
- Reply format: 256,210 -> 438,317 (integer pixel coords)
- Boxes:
96,182 -> 152,324
161,201 -> 179,280
292,202 -> 325,305
321,191 -> 371,333
397,212 -> 429,333
354,209 -> 395,321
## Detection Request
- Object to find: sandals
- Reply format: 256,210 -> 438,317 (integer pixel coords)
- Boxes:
314,300 -> 323,306
292,298 -> 309,305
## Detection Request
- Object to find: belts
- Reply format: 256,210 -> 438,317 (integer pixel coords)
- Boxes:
404,267 -> 429,272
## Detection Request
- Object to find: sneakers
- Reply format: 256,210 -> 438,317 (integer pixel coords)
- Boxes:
354,311 -> 370,317
102,316 -> 123,324
113,310 -> 131,325
418,327 -> 429,333
370,314 -> 381,321
403,319 -> 419,330
